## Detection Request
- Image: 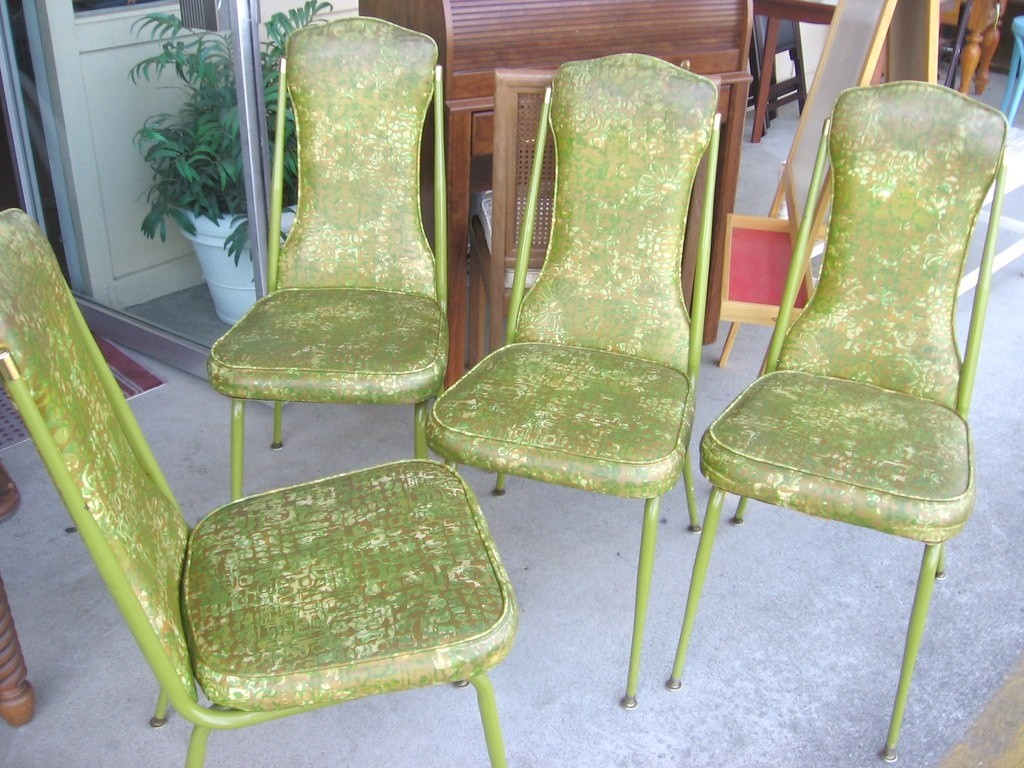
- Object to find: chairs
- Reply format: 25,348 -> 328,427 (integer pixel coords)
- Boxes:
206,16 -> 450,502
665,80 -> 1010,763
0,207 -> 520,768
424,52 -> 721,710
468,67 -> 754,365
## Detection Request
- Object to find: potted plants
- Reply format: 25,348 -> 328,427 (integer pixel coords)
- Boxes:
128,0 -> 333,325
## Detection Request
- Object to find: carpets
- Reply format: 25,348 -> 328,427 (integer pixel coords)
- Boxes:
0,330 -> 167,454
